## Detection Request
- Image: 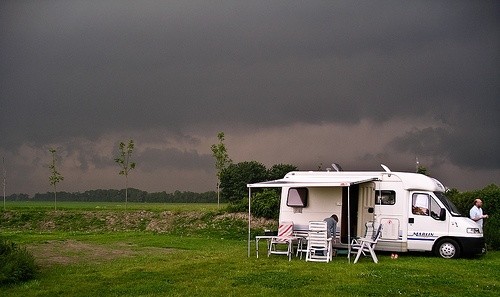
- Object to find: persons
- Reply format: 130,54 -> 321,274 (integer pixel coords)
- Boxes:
469,199 -> 489,230
323,214 -> 338,256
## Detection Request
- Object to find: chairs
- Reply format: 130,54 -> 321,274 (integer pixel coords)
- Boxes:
346,221 -> 382,264
266,221 -> 295,262
305,221 -> 333,264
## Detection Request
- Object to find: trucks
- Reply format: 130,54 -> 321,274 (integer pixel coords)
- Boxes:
246,163 -> 486,259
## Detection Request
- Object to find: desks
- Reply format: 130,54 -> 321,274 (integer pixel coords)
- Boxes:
255,235 -> 304,258
305,236 -> 341,260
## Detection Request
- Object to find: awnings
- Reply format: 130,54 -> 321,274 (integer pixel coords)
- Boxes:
245,177 -> 379,187
